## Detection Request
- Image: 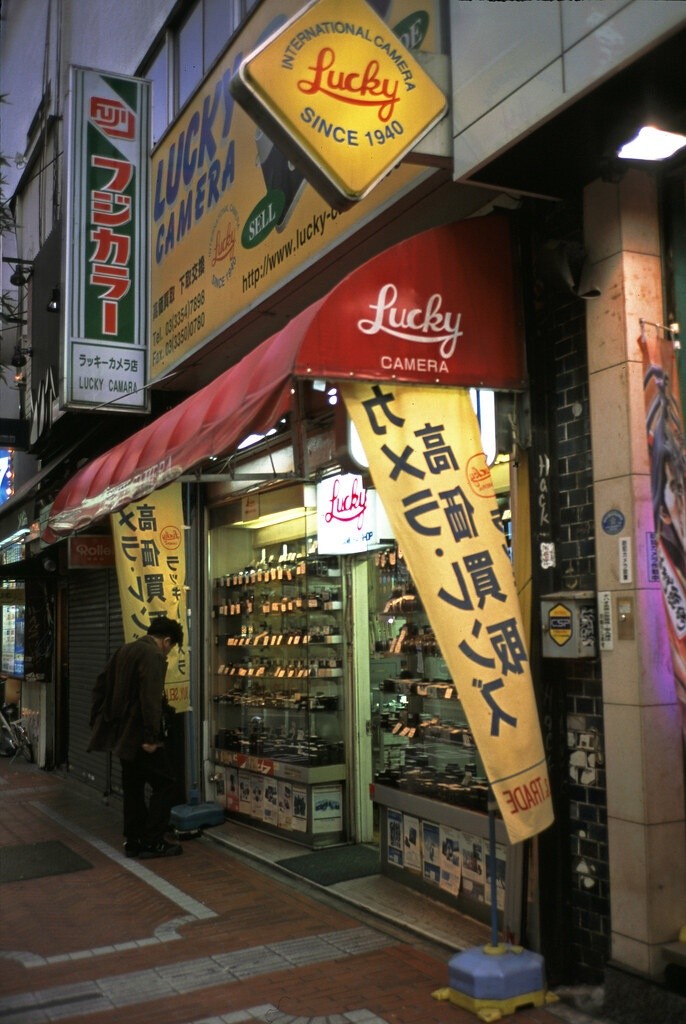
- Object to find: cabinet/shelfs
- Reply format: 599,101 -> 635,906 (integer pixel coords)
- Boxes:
215,547 -> 513,919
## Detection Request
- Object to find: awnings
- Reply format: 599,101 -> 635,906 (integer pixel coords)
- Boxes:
41,216 -> 534,551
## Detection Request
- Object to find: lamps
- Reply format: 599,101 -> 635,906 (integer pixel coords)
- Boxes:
46,280 -> 60,314
9,264 -> 34,286
11,345 -> 34,367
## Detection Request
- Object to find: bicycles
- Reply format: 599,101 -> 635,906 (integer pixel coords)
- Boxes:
0,680 -> 35,766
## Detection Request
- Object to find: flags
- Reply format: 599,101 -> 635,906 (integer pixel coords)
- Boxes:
110,481 -> 195,715
336,383 -> 556,846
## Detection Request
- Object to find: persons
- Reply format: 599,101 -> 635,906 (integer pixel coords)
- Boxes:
87,617 -> 185,860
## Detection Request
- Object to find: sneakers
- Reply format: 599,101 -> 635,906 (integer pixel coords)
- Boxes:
139,838 -> 183,858
125,837 -> 144,856
